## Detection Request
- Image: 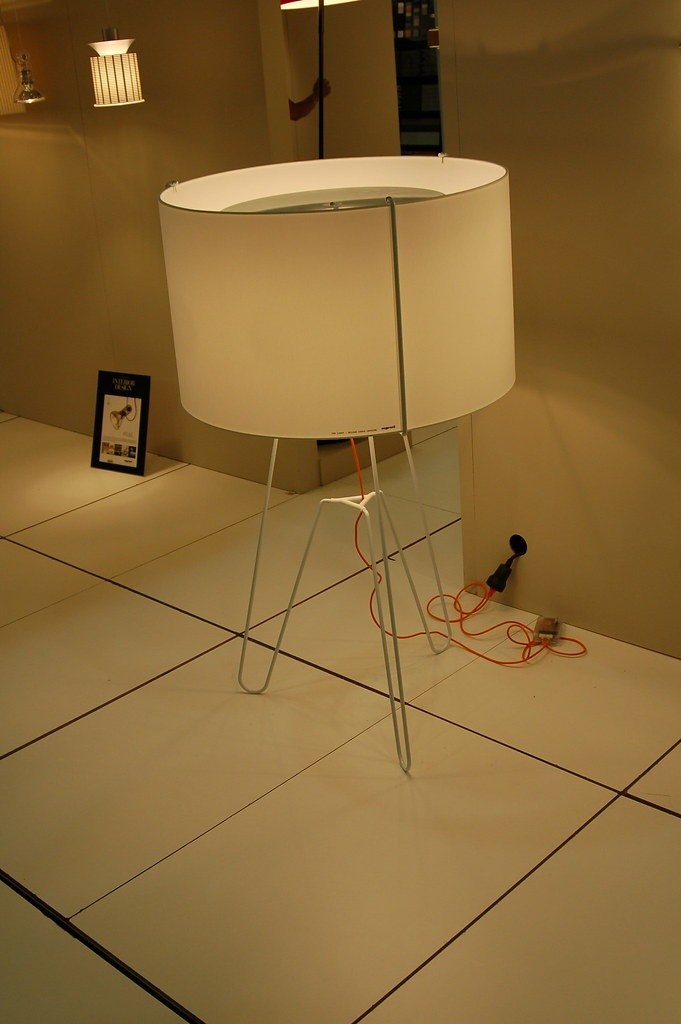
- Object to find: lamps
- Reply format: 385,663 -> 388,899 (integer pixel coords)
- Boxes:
13,1 -> 45,103
87,1 -> 151,107
158,155 -> 516,772
109,404 -> 134,430
